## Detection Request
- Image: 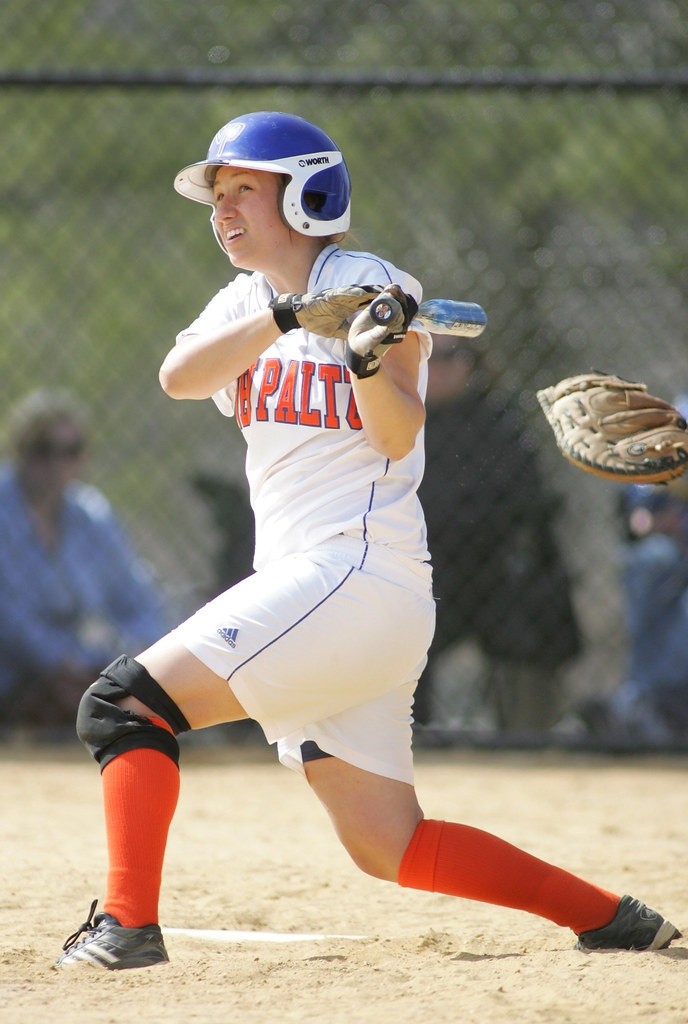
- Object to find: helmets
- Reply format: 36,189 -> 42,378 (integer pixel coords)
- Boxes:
174,111 -> 351,256
621,483 -> 672,539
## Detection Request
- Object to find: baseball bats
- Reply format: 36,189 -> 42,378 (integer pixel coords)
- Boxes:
370,300 -> 488,337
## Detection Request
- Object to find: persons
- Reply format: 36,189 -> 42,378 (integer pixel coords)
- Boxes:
1,336 -> 688,754
58,110 -> 682,969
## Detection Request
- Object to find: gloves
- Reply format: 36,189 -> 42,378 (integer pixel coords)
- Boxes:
271,283 -> 384,340
344,283 -> 418,379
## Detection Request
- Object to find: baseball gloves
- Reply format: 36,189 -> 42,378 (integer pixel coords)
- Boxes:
537,364 -> 688,481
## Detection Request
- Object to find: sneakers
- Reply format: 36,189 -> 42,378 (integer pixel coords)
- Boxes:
50,899 -> 170,971
574,894 -> 682,950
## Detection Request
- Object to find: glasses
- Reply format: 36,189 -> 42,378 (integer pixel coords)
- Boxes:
29,436 -> 83,458
